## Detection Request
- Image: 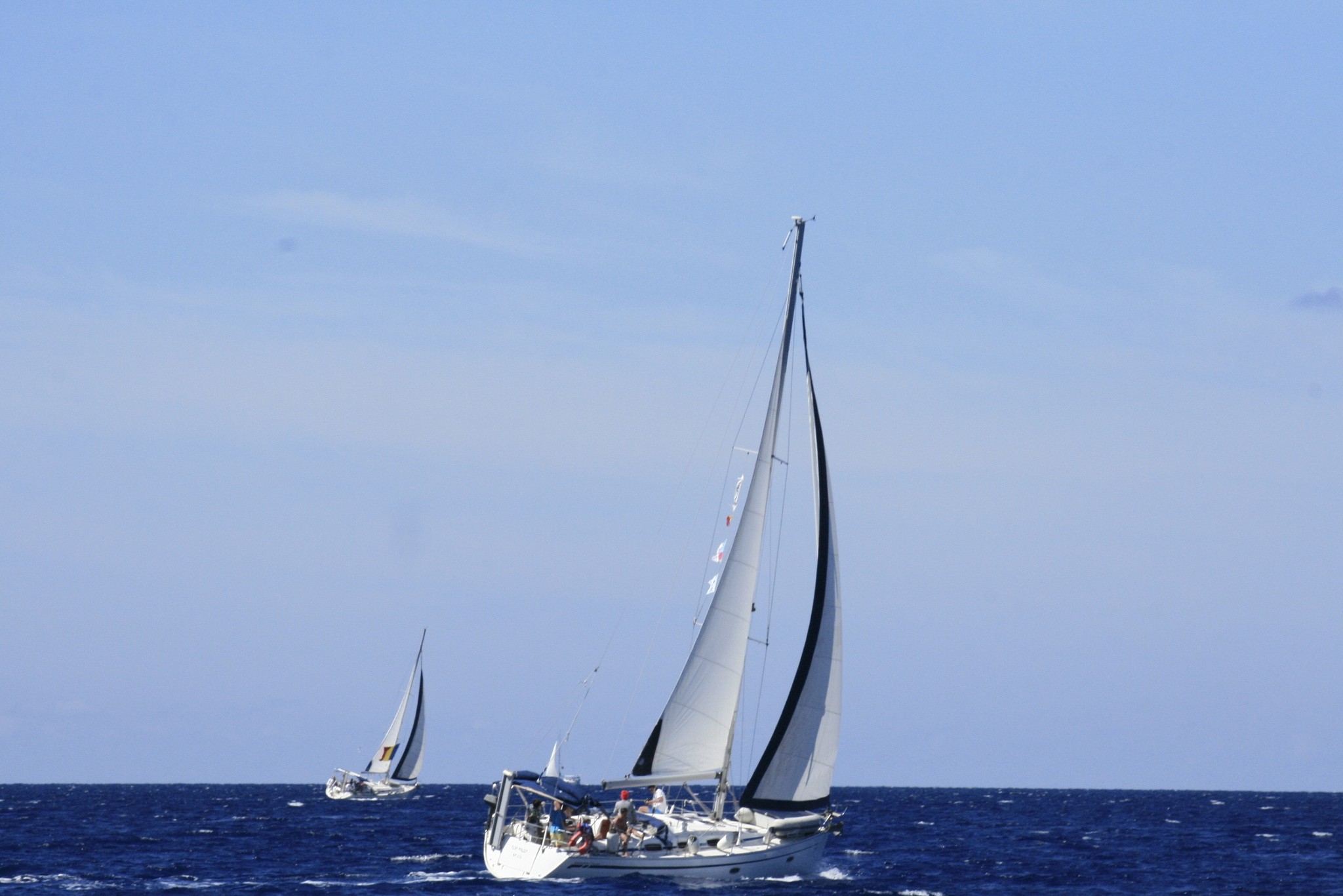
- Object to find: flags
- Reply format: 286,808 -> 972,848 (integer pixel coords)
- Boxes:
379,743 -> 400,761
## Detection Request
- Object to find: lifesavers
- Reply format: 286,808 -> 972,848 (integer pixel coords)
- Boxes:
568,831 -> 592,855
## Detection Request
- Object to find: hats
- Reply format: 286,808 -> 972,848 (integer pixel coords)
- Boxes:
621,790 -> 630,801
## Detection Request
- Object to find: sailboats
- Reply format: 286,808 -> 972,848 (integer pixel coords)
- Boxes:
481,216 -> 850,886
324,626 -> 430,805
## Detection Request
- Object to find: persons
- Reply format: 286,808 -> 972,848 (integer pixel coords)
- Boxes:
549,801 -> 566,847
639,785 -> 666,814
580,819 -> 594,842
345,778 -> 364,791
609,790 -> 643,857
